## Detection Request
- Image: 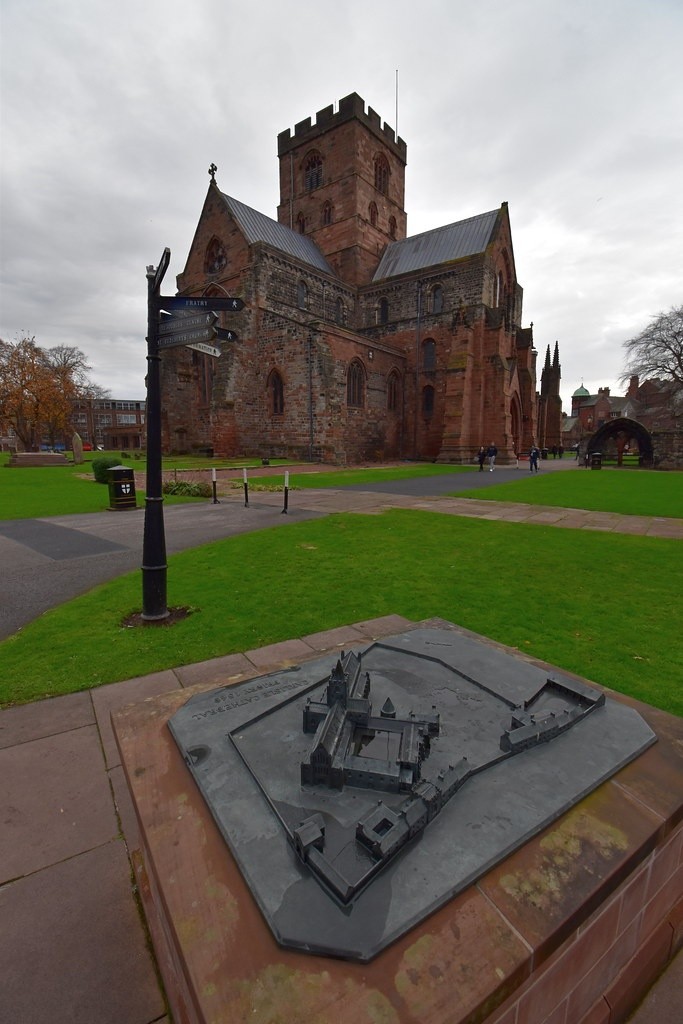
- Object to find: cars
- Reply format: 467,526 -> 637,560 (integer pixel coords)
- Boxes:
67,441 -> 94,452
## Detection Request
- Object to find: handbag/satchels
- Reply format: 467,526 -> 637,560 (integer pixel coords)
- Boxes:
474,457 -> 480,462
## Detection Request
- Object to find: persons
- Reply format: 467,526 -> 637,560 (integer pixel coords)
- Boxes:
552,444 -> 564,459
576,442 -> 580,461
528,446 -> 540,472
486,442 -> 497,472
477,446 -> 487,472
542,446 -> 549,459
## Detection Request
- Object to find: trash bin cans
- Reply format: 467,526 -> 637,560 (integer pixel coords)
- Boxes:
106,465 -> 137,508
541,448 -> 549,459
591,452 -> 602,470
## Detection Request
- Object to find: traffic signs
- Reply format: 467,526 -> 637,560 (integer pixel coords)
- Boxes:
156,310 -> 219,336
212,325 -> 239,342
158,296 -> 248,312
156,326 -> 219,351
184,342 -> 223,359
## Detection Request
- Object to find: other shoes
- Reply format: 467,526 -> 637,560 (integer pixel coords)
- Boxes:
490,468 -> 494,471
479,468 -> 483,472
529,470 -> 538,474
